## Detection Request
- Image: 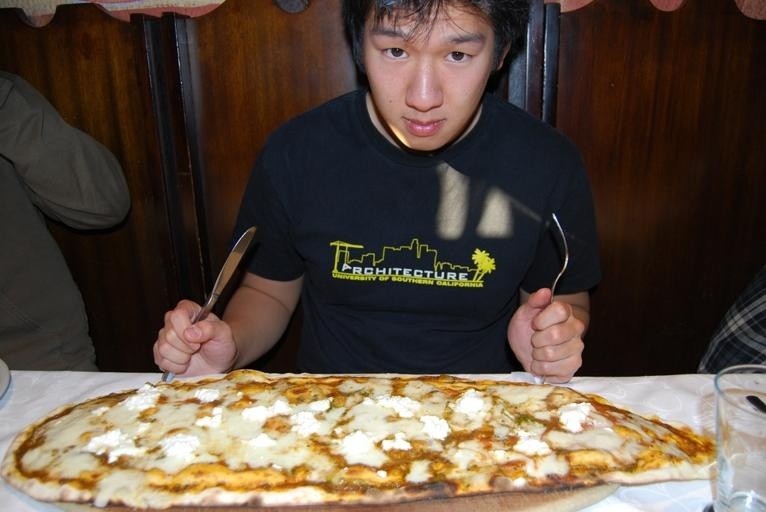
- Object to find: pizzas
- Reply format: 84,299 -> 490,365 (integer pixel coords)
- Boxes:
2,367 -> 725,511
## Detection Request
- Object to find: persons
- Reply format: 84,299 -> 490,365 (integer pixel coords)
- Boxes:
152,0 -> 609,384
692,257 -> 766,374
0,69 -> 133,370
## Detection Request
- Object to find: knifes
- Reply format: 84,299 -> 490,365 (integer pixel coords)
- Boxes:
159,227 -> 258,386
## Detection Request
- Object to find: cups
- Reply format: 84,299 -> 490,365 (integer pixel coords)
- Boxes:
708,363 -> 766,511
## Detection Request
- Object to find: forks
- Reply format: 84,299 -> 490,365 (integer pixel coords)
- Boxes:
530,211 -> 574,387
